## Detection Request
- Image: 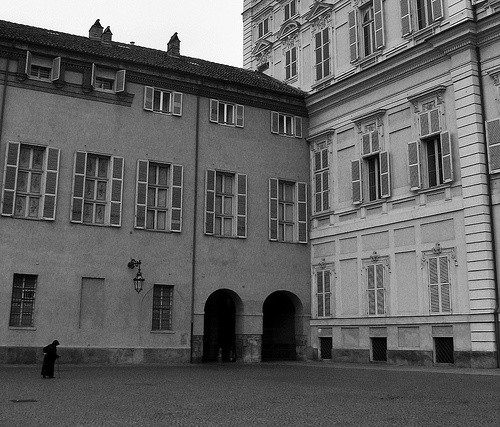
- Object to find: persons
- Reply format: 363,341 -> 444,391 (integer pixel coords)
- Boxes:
40,340 -> 61,379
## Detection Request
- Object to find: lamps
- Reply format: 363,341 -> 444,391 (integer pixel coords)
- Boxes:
127,258 -> 145,294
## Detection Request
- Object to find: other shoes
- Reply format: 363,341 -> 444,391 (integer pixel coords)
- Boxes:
43,376 -> 56,379
